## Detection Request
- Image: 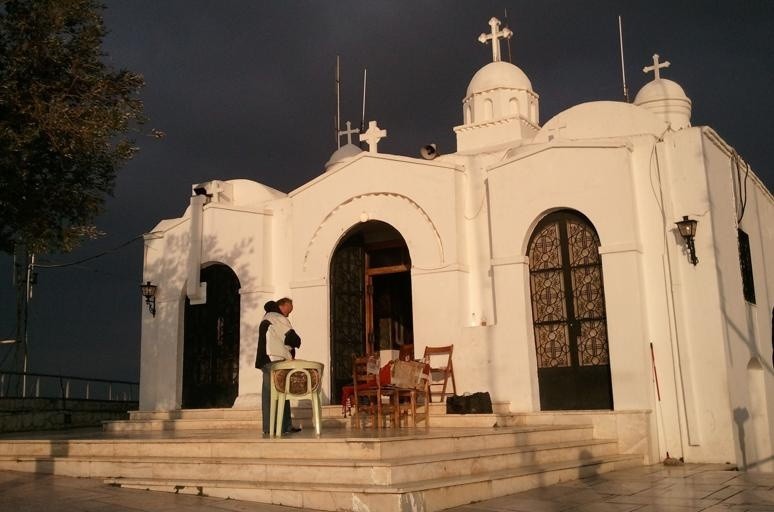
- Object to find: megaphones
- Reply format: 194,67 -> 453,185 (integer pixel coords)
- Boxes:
420,143 -> 438,160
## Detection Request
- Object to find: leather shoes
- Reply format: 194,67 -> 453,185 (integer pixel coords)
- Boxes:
282,427 -> 302,434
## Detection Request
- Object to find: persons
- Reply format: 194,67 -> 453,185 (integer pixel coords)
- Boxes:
256,298 -> 302,432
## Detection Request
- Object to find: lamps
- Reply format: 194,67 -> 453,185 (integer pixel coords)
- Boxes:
675,215 -> 698,266
141,281 -> 157,318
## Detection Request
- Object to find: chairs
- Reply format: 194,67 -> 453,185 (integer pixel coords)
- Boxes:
270,359 -> 324,436
343,344 -> 458,430
